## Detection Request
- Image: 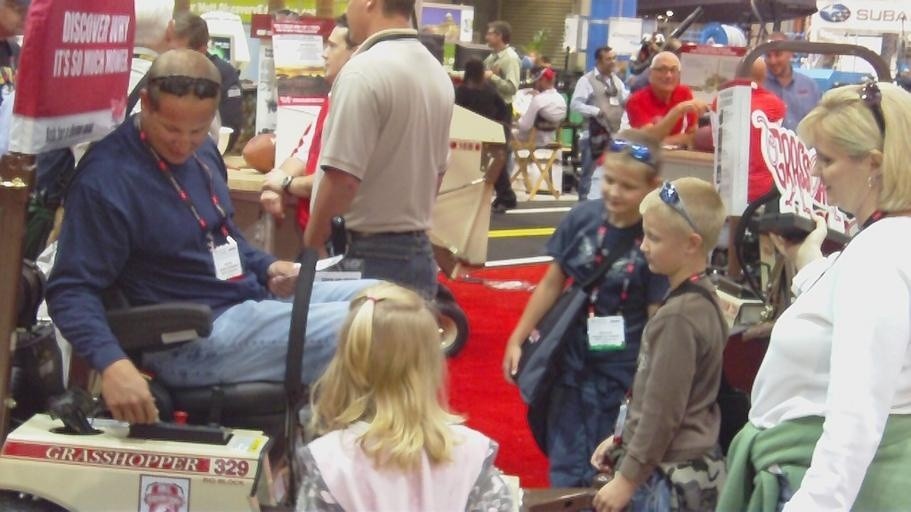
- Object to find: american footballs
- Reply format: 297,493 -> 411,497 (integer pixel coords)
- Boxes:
243,134 -> 275,173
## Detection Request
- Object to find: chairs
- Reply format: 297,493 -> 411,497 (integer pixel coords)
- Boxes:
508,113 -> 568,203
61,167 -> 309,456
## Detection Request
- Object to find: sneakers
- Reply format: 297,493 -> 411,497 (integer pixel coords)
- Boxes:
491,192 -> 518,213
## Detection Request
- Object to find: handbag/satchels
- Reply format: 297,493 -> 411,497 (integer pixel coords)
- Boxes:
505,257 -> 617,410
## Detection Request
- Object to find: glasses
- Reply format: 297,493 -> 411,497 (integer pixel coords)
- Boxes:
858,81 -> 886,130
660,180 -> 699,231
147,72 -> 219,99
608,137 -> 657,168
653,66 -> 680,74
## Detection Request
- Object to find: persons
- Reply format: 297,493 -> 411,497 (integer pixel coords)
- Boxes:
511,30 -> 821,201
504,81 -> 910,512
1,1 -> 521,512
